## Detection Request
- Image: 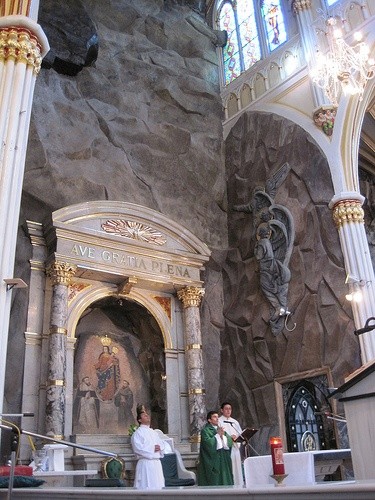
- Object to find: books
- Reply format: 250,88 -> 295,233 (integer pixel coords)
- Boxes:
233,428 -> 259,444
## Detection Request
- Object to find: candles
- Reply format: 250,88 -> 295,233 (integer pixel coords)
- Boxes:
270,437 -> 284,475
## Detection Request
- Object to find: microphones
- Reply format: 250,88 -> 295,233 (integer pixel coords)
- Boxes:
314,412 -> 330,416
224,421 -> 234,423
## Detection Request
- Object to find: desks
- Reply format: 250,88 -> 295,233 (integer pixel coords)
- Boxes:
32,470 -> 98,476
244,449 -> 356,487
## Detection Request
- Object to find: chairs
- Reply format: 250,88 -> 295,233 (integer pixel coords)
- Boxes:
300,430 -> 317,452
153,429 -> 197,489
101,457 -> 135,487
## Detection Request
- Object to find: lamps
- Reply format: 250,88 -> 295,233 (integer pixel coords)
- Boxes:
307,8 -> 375,103
279,307 -> 296,331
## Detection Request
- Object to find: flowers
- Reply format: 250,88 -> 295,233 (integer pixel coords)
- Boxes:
128,424 -> 139,436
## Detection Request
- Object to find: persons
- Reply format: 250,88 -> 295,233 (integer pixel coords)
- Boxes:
233,162 -> 297,331
198,411 -> 233,486
218,402 -> 244,485
131,405 -> 165,490
